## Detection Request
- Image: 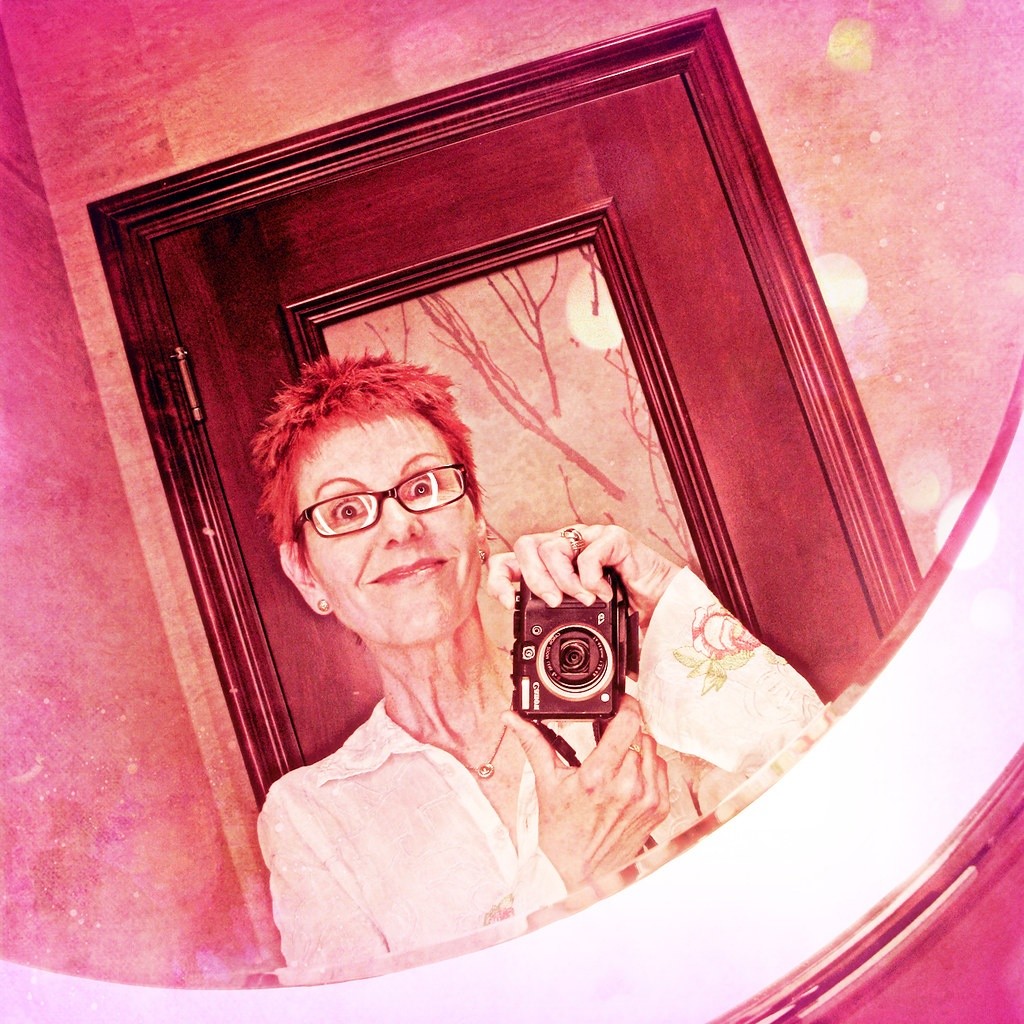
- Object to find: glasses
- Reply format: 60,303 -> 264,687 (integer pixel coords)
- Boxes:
287,463 -> 470,540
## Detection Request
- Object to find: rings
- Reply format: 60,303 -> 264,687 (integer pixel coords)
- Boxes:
630,742 -> 644,758
561,528 -> 585,555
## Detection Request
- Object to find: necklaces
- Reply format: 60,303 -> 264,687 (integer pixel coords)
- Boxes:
464,725 -> 508,778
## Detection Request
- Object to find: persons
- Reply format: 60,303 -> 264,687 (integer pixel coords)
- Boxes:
256,352 -> 827,986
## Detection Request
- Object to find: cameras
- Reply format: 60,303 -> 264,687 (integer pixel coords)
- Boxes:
511,572 -> 628,719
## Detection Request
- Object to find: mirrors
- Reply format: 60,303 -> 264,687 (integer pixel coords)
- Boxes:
0,0 -> 1024,1024
